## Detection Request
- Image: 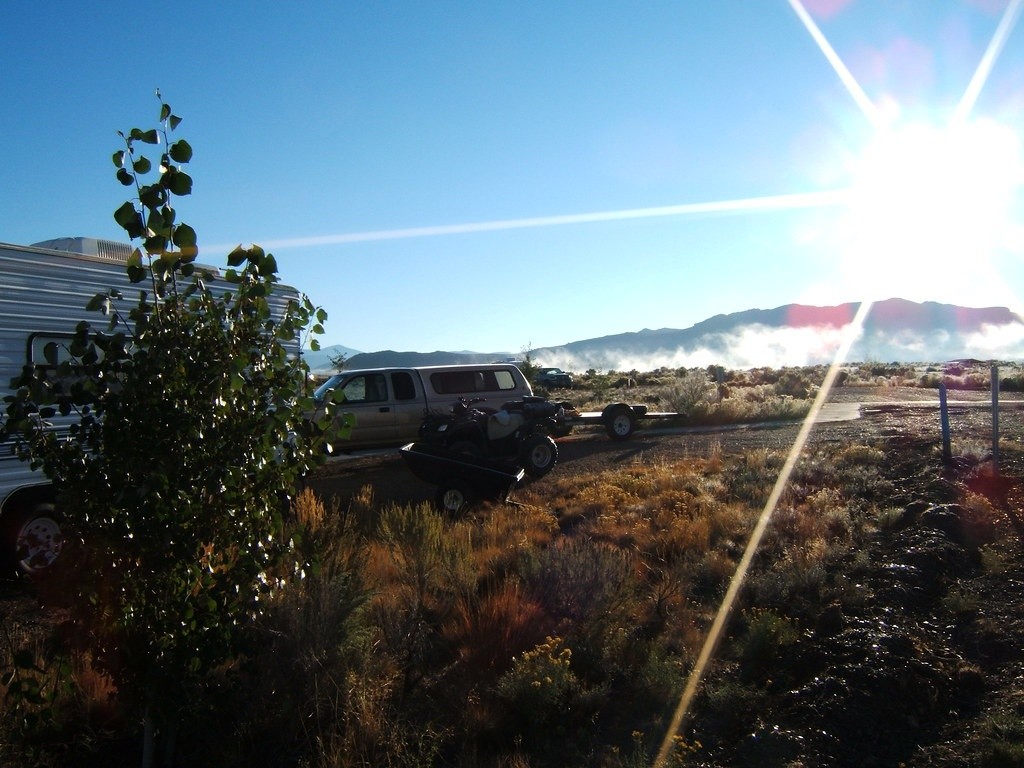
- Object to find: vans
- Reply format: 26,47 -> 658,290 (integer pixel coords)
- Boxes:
301,364 -> 533,453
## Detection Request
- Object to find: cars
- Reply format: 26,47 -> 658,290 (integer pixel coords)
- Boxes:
536,368 -> 573,388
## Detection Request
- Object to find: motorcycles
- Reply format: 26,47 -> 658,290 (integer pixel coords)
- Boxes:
418,396 -> 566,480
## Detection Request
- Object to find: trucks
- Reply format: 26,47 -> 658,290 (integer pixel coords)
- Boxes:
0,238 -> 301,584
